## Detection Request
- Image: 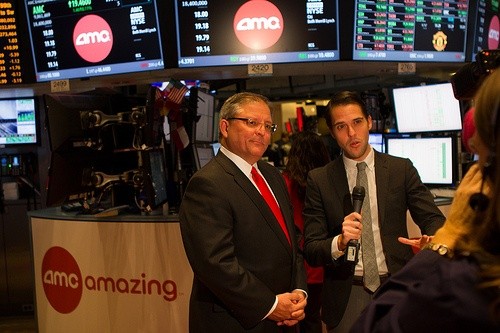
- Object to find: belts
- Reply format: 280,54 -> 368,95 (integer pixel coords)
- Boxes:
353,275 -> 391,284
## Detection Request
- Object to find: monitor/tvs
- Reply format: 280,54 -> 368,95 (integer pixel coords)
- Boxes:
367,80 -> 464,189
0,88 -> 41,147
0,0 -> 500,87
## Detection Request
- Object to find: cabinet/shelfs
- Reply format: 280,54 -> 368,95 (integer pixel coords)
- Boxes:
0,151 -> 41,216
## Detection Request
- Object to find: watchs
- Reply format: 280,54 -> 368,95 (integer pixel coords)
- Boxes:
421,243 -> 455,260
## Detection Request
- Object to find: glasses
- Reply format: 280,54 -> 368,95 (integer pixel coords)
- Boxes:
226,117 -> 273,131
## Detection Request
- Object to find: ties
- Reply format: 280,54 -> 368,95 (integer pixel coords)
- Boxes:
251,166 -> 292,246
355,162 -> 381,293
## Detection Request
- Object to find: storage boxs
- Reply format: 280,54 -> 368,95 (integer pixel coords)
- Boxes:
3,182 -> 20,201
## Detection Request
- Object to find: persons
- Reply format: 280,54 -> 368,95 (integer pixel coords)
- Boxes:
303,92 -> 446,333
282,132 -> 332,333
179,92 -> 308,333
350,66 -> 500,333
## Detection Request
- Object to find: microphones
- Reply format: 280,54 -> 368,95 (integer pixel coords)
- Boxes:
350,185 -> 365,246
